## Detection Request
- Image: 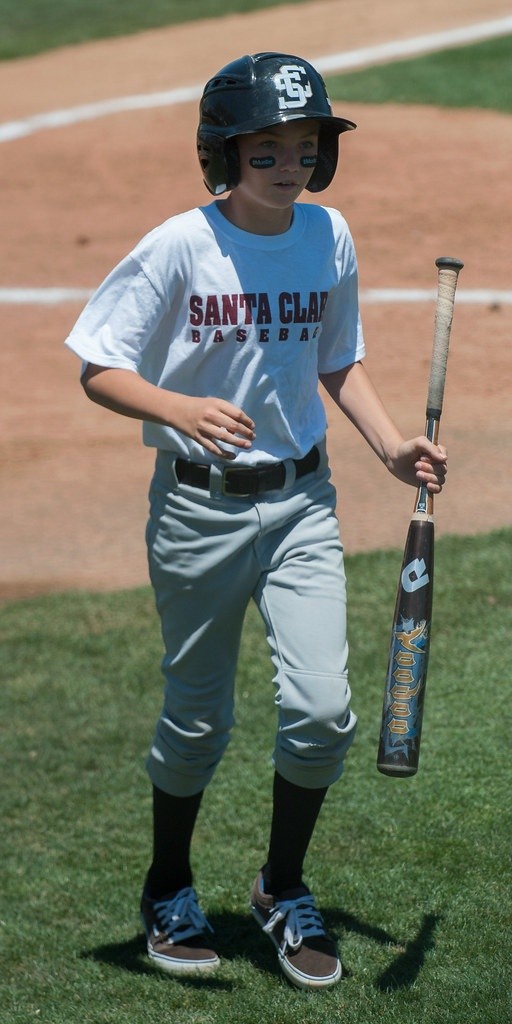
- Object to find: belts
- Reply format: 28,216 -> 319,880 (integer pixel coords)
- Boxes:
175,445 -> 319,498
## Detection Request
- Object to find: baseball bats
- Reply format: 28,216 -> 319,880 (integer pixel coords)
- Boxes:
371,253 -> 469,779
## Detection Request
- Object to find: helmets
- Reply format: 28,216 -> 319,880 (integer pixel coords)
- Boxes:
197,52 -> 357,197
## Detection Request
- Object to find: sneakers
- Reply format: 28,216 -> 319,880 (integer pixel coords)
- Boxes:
250,864 -> 342,992
141,864 -> 221,980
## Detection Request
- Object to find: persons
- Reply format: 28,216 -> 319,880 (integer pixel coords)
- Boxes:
62,51 -> 450,989
